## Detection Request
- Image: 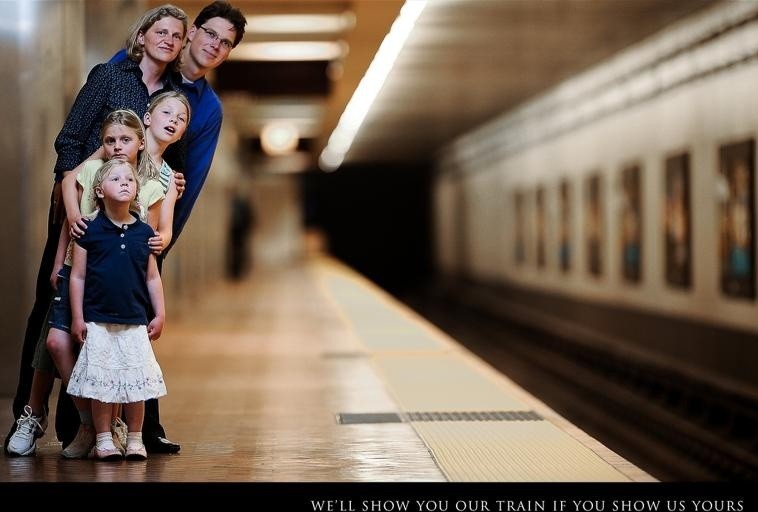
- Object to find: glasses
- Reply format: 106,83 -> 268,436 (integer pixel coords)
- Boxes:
200,26 -> 232,49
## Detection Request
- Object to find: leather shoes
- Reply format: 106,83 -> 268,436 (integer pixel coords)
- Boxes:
146,437 -> 180,453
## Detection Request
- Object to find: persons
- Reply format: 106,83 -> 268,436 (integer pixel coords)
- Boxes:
44,107 -> 167,462
54,1 -> 249,453
6,89 -> 192,459
3,4 -> 189,459
69,158 -> 165,462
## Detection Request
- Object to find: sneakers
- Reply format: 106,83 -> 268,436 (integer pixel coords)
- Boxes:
4,404 -> 49,458
61,416 -> 147,460
